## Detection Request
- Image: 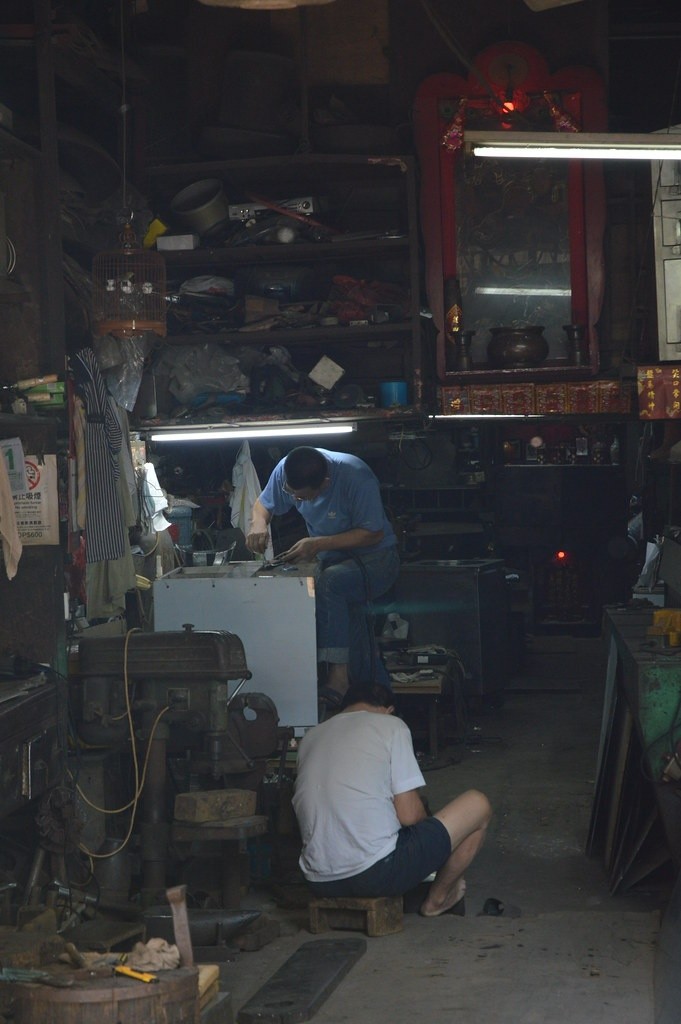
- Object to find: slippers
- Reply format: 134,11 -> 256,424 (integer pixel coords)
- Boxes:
478,898 -> 521,916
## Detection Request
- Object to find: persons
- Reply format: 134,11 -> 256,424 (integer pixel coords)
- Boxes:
290,682 -> 491,918
247,444 -> 402,699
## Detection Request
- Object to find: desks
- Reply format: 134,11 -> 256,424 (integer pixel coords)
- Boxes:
388,660 -> 447,760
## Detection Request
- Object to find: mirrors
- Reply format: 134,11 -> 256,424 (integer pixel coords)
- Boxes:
438,93 -> 589,379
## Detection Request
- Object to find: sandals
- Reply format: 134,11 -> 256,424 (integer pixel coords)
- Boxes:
318,684 -> 344,710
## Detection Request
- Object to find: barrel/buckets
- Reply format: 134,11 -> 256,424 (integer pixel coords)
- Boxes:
377,381 -> 409,410
169,179 -> 233,244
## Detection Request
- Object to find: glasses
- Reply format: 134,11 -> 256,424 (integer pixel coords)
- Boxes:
281,481 -> 321,503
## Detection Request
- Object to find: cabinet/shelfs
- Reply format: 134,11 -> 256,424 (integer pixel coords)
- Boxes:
133,152 -> 423,426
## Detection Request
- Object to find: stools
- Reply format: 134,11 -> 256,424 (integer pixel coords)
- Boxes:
308,897 -> 404,937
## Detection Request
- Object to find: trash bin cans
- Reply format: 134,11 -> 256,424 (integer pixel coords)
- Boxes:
395,559 -> 509,697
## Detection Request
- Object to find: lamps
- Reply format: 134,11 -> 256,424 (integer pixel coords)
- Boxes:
144,421 -> 358,441
459,126 -> 681,161
